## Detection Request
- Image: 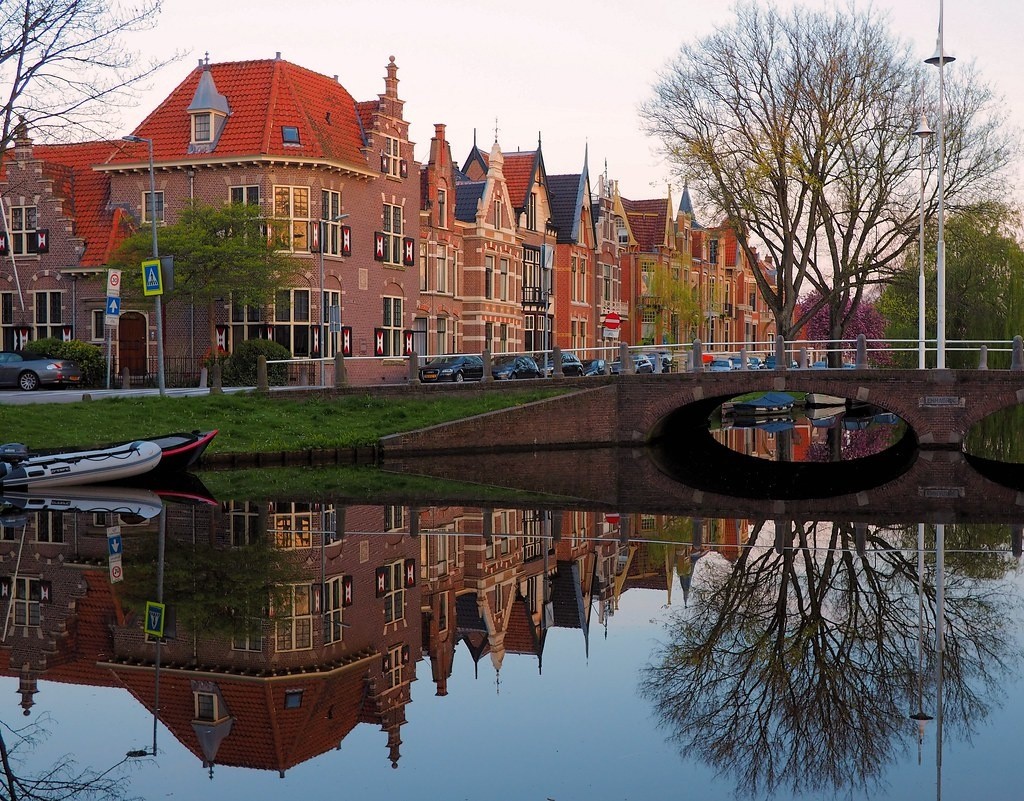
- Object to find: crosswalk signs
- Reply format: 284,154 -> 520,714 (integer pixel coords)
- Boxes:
141,260 -> 163,296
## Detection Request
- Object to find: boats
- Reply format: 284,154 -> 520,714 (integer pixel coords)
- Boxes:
733,393 -> 795,411
0,473 -> 219,527
0,429 -> 220,488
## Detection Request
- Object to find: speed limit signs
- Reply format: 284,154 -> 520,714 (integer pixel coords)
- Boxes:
110,274 -> 119,286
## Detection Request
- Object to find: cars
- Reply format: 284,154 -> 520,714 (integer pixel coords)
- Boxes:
581,360 -> 607,376
609,354 -> 653,375
709,357 -> 762,371
765,356 -> 855,369
0,351 -> 83,391
418,354 -> 483,383
492,355 -> 540,380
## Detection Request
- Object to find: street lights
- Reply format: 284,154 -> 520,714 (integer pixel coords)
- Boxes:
912,107 -> 935,369
122,136 -> 169,396
924,0 -> 956,368
320,213 -> 349,385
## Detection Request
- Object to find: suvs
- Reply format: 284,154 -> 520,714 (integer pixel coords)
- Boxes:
533,352 -> 584,378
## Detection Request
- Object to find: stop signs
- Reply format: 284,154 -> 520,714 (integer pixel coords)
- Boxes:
605,313 -> 620,329
